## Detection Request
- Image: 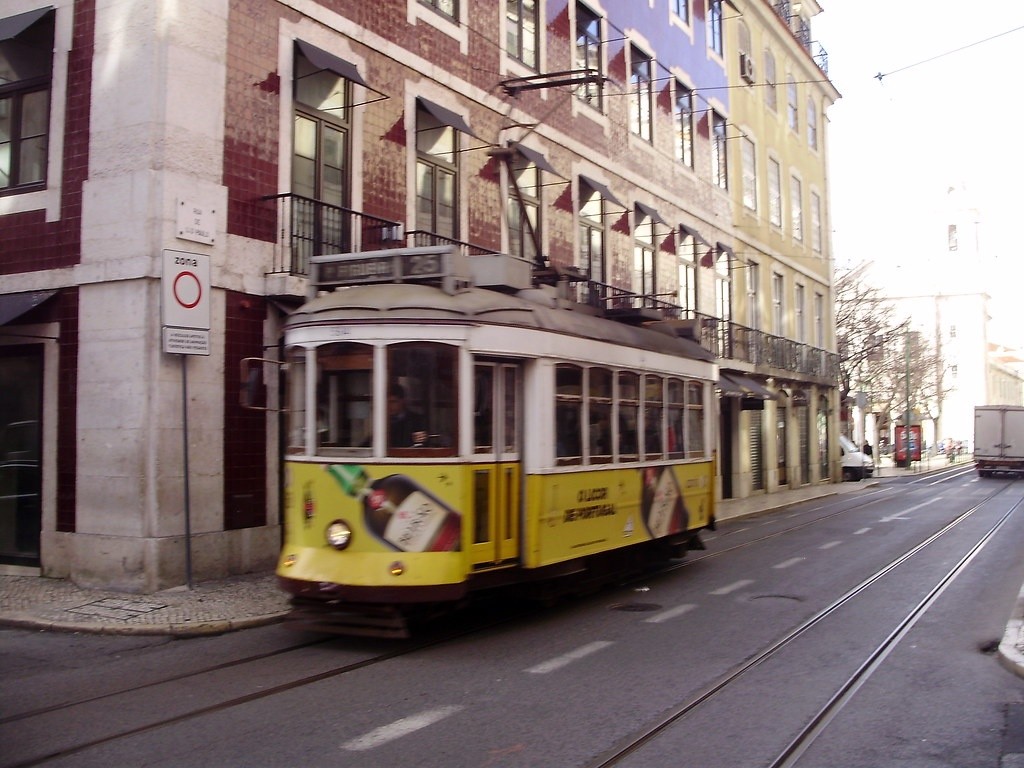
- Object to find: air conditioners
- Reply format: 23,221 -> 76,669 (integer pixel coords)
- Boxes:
741,54 -> 756,85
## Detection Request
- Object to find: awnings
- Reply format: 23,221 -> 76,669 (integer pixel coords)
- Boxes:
718,242 -> 739,262
512,142 -> 573,189
680,224 -> 718,254
0,290 -> 59,327
579,174 -> 633,217
718,373 -> 778,401
296,38 -> 390,97
0,5 -> 55,41
418,96 -> 492,145
635,202 -> 680,236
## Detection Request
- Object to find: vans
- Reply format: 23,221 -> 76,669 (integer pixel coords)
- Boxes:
841,433 -> 874,484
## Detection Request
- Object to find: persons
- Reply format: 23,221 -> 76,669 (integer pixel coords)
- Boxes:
864,440 -> 872,455
359,384 -> 429,448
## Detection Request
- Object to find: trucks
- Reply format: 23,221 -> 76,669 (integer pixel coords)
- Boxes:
974,404 -> 1024,477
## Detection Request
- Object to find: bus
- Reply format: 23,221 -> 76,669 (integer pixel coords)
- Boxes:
272,247 -> 723,640
